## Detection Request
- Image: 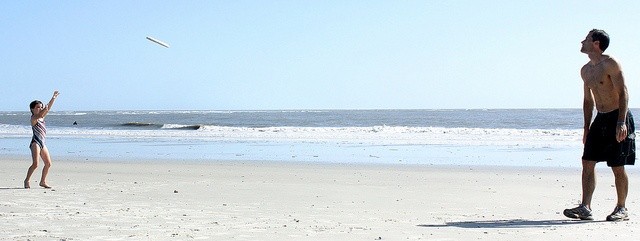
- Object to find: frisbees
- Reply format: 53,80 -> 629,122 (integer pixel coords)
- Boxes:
146,36 -> 169,48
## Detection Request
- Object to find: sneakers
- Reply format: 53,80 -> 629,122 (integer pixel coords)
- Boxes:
564,202 -> 593,220
607,206 -> 629,221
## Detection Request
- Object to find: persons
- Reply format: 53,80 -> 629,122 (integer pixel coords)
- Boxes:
23,90 -> 61,189
562,28 -> 629,222
73,121 -> 77,126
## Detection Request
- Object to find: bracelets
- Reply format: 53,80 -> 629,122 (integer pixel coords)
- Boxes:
617,121 -> 626,125
